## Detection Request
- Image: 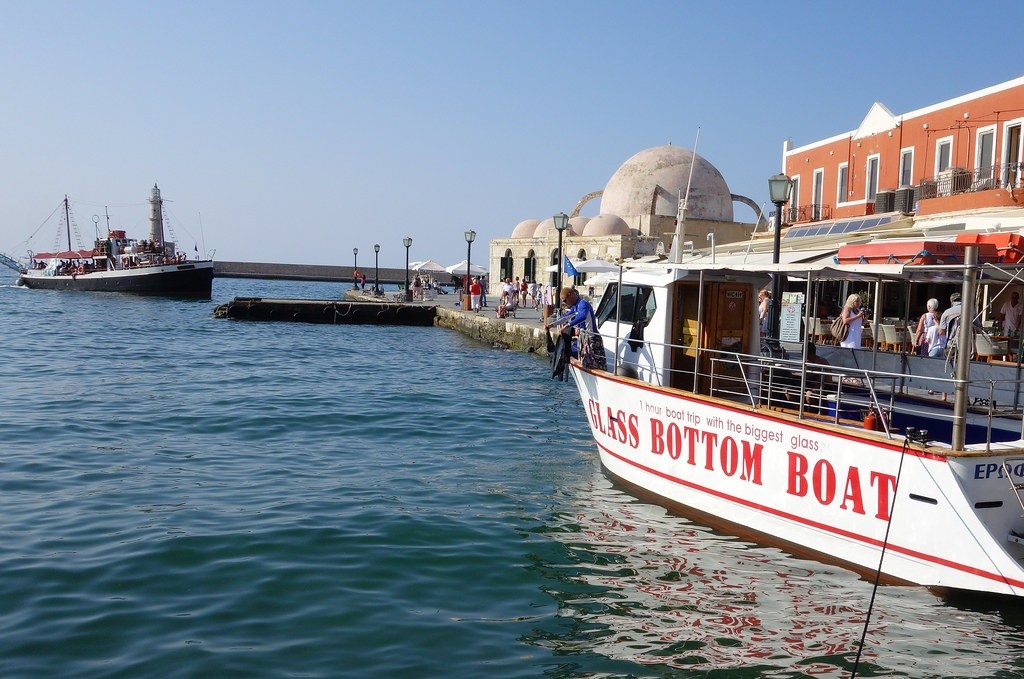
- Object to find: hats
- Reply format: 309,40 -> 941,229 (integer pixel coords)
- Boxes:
949,292 -> 962,301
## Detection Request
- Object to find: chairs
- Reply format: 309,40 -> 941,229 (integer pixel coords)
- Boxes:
802,315 -> 1008,364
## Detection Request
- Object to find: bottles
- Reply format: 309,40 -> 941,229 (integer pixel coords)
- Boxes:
994,321 -> 999,335
1008,326 -> 1020,338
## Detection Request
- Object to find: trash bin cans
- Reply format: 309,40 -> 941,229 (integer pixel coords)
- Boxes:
409,290 -> 413,302
544,305 -> 553,328
463,294 -> 471,311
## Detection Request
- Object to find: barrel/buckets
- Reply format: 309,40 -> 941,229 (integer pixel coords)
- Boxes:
827,393 -> 867,420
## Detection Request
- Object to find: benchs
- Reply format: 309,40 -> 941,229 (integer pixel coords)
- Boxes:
505,299 -> 519,317
362,287 -> 371,296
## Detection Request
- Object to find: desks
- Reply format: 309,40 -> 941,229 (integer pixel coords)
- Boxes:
895,327 -> 909,353
819,320 -> 835,345
990,335 -> 1016,362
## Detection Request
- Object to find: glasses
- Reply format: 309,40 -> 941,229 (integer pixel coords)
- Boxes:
562,297 -> 568,303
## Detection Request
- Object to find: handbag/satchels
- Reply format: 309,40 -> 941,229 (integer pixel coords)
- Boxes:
829,316 -> 850,342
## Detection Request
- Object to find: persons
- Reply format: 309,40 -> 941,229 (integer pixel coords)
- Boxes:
125,238 -> 186,267
545,287 -> 608,371
495,275 -> 544,320
59,259 -> 95,279
757,290 -> 772,337
470,275 -> 489,312
998,291 -> 1024,337
33,259 -> 48,269
412,274 -> 439,299
914,292 -> 982,360
360,274 -> 366,289
839,293 -> 870,348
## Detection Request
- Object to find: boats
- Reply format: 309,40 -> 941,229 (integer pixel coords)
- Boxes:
1,182 -> 216,302
557,128 -> 1023,598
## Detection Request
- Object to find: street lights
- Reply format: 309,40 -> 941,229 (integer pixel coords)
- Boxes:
372,243 -> 382,298
401,236 -> 416,303
352,247 -> 361,290
553,211 -> 570,315
459,230 -> 477,312
755,171 -> 798,373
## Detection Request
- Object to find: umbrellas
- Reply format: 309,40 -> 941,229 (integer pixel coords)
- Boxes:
446,259 -> 488,276
576,257 -> 627,275
409,259 -> 446,283
584,271 -> 623,287
544,258 -> 586,273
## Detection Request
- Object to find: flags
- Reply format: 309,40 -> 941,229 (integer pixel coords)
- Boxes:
564,255 -> 579,277
